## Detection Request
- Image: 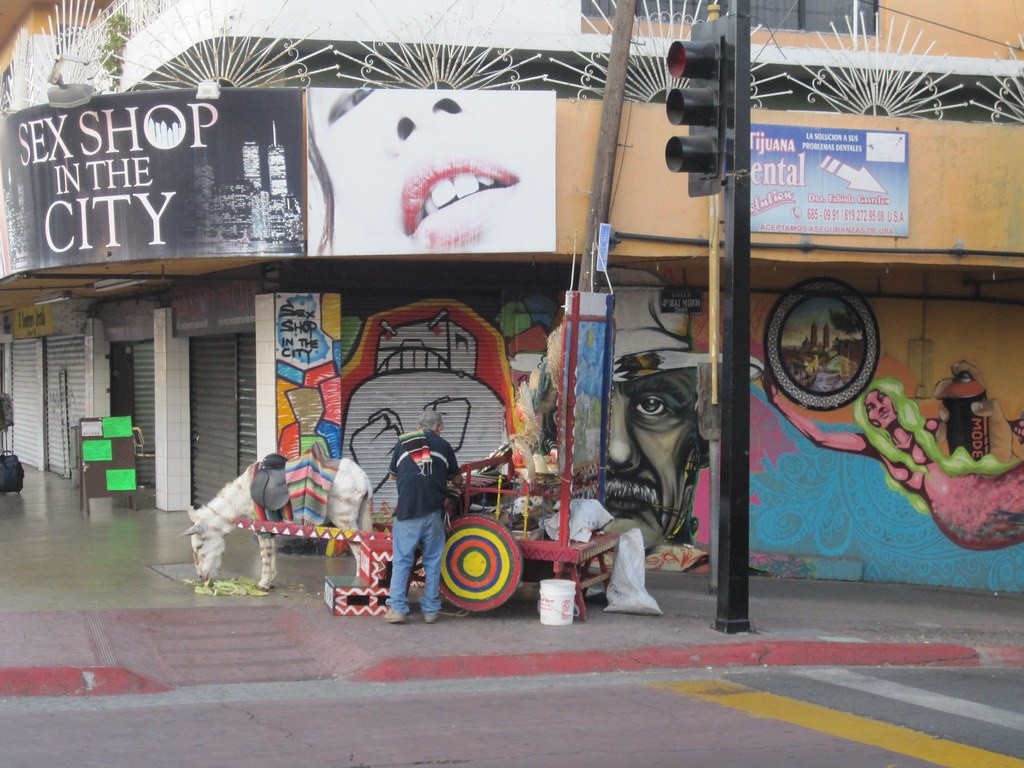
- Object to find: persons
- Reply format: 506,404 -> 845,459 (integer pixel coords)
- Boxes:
305,87 -> 556,251
0,393 -> 9,433
384,411 -> 463,623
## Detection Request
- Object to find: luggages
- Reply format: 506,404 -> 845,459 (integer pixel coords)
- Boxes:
0,428 -> 25,494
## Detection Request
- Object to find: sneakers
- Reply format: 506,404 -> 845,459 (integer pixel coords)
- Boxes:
384,609 -> 404,622
423,615 -> 438,623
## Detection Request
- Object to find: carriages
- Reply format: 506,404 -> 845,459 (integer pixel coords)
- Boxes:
173,438 -> 624,614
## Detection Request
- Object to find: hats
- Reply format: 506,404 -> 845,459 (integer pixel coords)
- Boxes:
515,453 -> 561,487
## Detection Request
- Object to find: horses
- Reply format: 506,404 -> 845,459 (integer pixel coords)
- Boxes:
177,454 -> 371,592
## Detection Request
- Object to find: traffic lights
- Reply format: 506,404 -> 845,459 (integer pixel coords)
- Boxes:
664,14 -> 725,197
